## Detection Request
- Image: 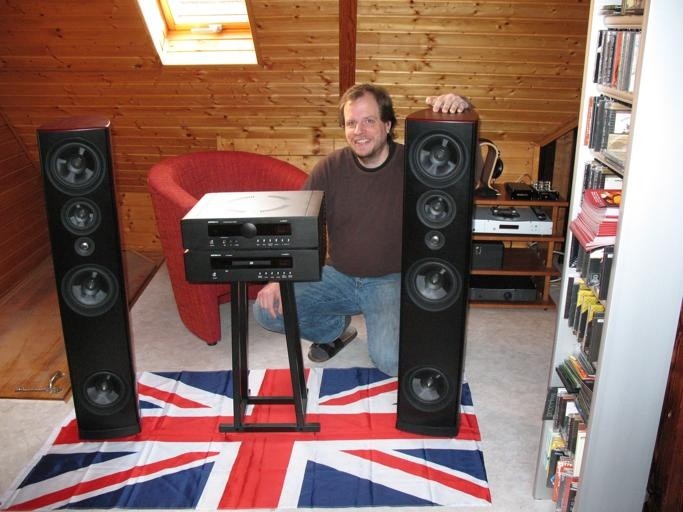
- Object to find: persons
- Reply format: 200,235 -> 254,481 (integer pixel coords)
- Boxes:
251,80 -> 486,377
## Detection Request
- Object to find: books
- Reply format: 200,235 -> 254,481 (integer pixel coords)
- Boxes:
569,162 -> 623,251
542,237 -> 617,512
592,1 -> 646,93
584,96 -> 631,174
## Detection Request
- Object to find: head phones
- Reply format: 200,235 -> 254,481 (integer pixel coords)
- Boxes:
480,138 -> 504,179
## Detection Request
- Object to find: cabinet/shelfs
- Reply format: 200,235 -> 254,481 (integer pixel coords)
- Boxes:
471,185 -> 568,311
531,0 -> 683,512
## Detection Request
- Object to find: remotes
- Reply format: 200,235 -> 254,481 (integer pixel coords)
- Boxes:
532,206 -> 546,220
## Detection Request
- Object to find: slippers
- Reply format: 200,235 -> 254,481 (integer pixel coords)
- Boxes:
308,324 -> 358,363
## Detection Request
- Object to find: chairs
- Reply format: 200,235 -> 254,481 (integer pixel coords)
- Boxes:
147,149 -> 310,343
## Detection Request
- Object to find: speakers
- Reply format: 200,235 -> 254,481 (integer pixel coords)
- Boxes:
35,120 -> 142,440
396,105 -> 479,436
